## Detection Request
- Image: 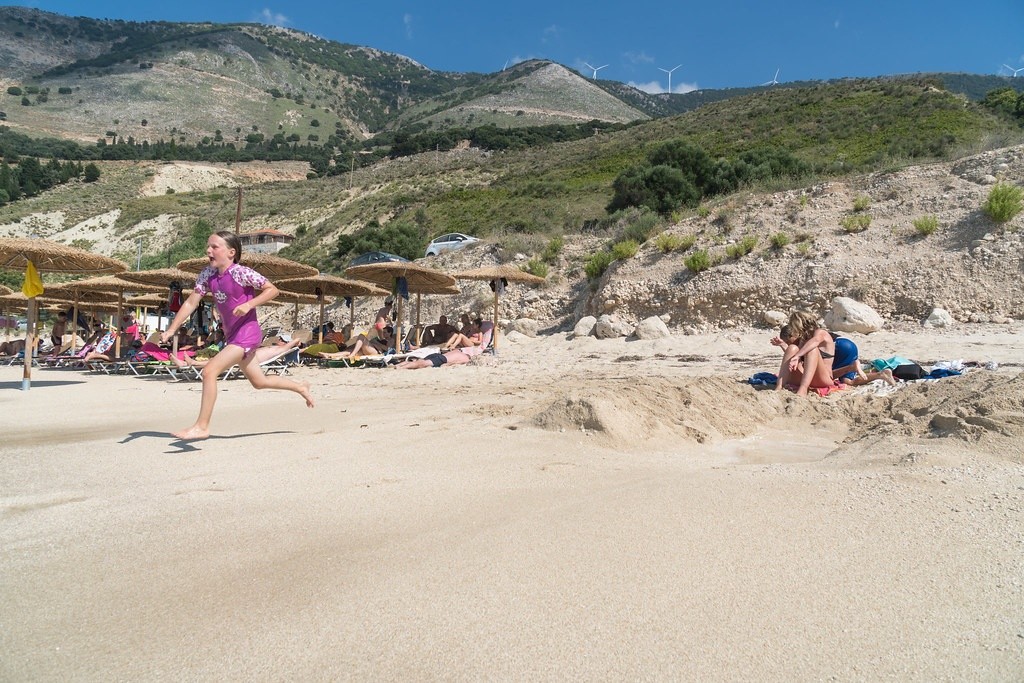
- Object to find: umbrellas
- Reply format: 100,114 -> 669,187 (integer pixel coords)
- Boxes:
409,282 -> 459,336
340,281 -> 392,336
450,265 -> 545,353
270,273 -> 372,344
343,259 -> 456,355
0,238 -> 132,391
0,253 -> 319,351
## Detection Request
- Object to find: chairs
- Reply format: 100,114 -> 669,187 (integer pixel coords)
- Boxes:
0,321 -> 495,380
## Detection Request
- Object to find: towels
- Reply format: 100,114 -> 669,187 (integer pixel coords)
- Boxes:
871,356 -> 914,371
136,342 -> 197,363
396,275 -> 410,300
785,381 -> 845,397
925,369 -> 961,379
749,371 -> 778,384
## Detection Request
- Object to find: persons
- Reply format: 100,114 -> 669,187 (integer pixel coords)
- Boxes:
767,312 -> 837,395
79,319 -> 494,368
86,315 -> 105,346
779,324 -> 898,387
0,338 -> 45,355
158,230 -> 313,439
50,311 -> 69,360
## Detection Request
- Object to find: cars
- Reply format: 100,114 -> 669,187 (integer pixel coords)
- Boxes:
348,251 -> 411,267
424,232 -> 481,258
